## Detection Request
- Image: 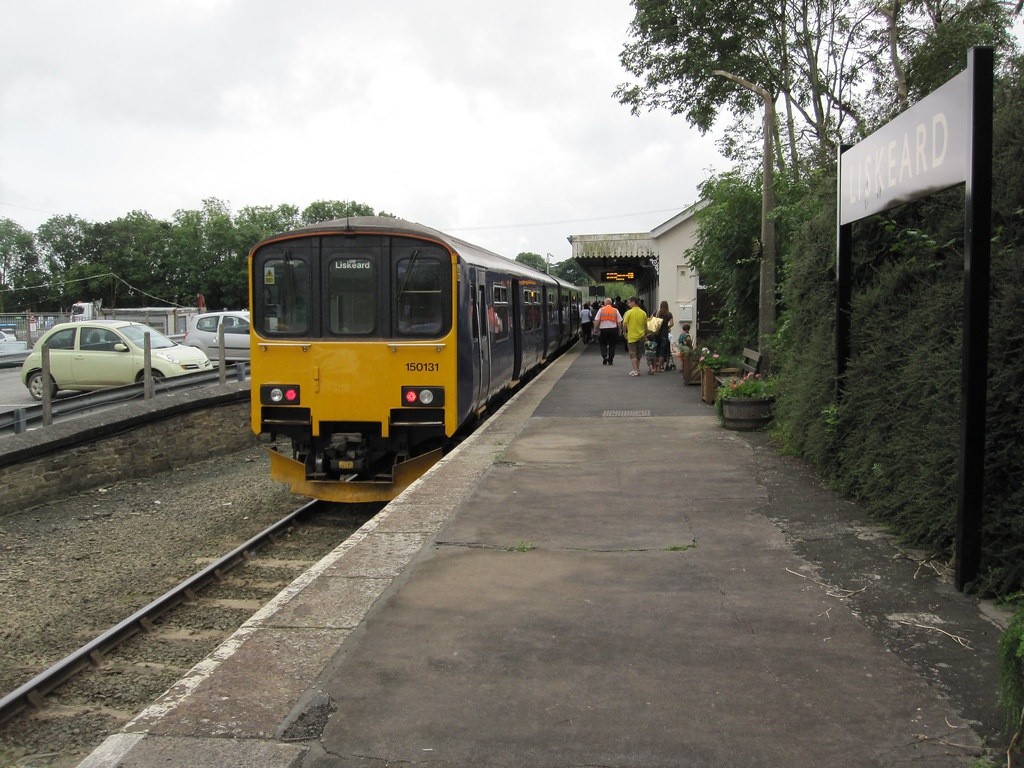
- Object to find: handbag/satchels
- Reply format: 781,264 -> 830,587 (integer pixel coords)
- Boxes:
646,308 -> 664,335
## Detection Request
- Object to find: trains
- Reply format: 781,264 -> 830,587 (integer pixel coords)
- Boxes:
248,217 -> 582,505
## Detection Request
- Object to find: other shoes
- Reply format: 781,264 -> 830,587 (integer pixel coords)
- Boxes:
648,370 -> 655,375
603,357 -> 608,364
629,369 -> 640,376
607,358 -> 613,365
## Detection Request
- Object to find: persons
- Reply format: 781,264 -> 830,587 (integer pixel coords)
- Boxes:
667,329 -> 674,351
579,295 -> 647,353
594,298 -> 624,365
623,296 -> 648,377
644,334 -> 658,375
651,300 -> 674,372
678,324 -> 694,371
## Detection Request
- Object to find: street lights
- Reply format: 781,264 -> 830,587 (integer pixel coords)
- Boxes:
546,252 -> 555,274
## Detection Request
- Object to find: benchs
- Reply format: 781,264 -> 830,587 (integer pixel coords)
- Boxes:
714,346 -> 763,387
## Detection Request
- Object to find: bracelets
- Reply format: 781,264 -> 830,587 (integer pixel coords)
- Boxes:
619,330 -> 622,332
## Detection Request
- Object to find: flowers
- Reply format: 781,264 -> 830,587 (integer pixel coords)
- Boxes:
714,372 -> 776,426
699,353 -> 729,371
680,347 -> 718,360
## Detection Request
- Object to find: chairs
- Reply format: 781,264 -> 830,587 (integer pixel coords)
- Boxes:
91,332 -> 120,350
226,317 -> 249,334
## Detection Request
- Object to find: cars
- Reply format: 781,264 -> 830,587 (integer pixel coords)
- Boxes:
21,318 -> 212,403
0,330 -> 17,343
181,311 -> 253,369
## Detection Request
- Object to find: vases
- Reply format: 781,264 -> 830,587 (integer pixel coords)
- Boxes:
701,366 -> 742,402
723,395 -> 776,430
682,355 -> 704,385
